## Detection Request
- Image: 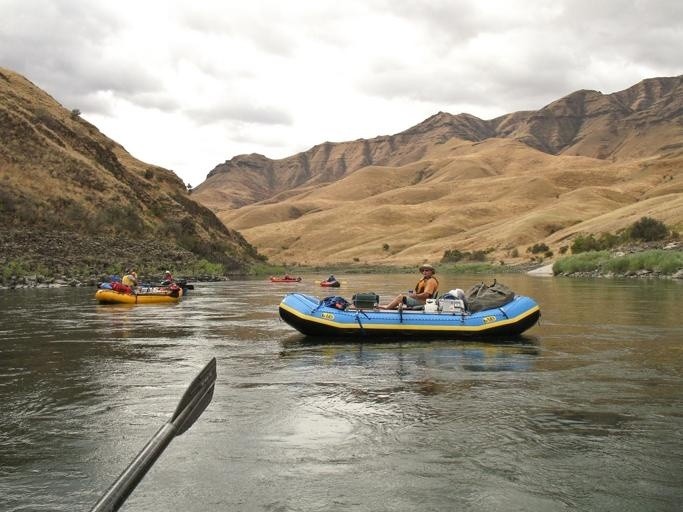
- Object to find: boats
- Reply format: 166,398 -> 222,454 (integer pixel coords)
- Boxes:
269,275 -> 302,282
278,291 -> 541,342
95,286 -> 183,304
320,280 -> 340,287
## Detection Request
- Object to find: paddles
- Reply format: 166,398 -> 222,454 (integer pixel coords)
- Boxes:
164,285 -> 194,290
89,357 -> 216,511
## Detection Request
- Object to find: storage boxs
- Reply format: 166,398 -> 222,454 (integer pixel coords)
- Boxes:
354,292 -> 380,309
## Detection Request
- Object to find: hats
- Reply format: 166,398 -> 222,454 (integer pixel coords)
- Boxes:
419,263 -> 436,276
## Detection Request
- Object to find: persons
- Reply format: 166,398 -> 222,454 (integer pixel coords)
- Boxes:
327,274 -> 336,282
374,263 -> 439,310
120,270 -> 137,290
158,270 -> 171,285
